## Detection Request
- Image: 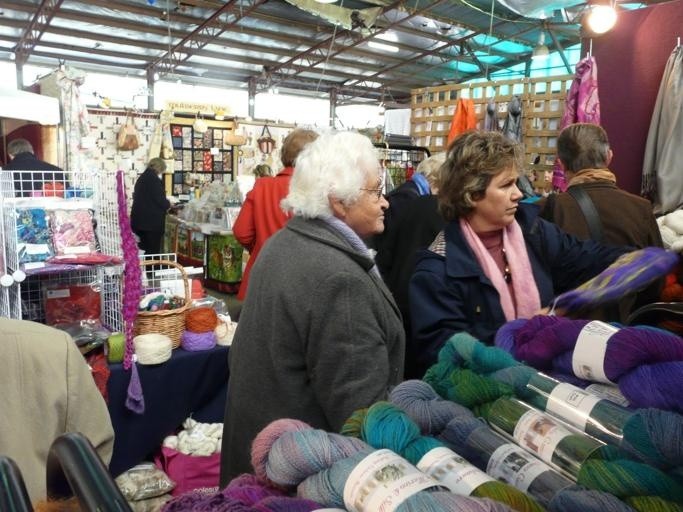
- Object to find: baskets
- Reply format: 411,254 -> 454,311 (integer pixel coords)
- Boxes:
118,259 -> 190,350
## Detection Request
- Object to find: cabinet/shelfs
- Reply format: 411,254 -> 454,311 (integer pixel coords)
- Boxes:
2,169 -> 127,356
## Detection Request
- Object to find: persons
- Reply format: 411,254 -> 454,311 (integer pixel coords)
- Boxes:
528,122 -> 667,324
232,129 -> 320,302
0,314 -> 116,508
407,131 -> 640,380
217,129 -> 406,493
377,149 -> 446,281
131,156 -> 176,275
2,139 -> 70,197
254,164 -> 275,180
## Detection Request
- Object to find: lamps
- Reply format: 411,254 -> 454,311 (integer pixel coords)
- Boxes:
531,28 -> 549,59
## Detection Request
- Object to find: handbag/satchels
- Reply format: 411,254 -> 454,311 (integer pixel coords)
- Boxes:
258,137 -> 275,154
117,124 -> 139,151
193,120 -> 207,132
226,135 -> 246,145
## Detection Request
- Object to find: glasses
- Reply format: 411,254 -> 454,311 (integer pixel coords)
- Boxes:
360,185 -> 386,200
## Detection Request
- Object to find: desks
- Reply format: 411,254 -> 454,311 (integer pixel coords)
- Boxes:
84,346 -> 232,476
166,213 -> 252,293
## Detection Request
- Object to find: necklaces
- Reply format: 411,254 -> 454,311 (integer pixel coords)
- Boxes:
501,245 -> 512,283
